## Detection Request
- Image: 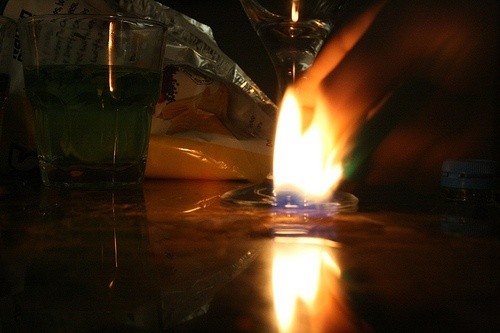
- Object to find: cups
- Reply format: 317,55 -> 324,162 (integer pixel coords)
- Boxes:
14,14 -> 169,191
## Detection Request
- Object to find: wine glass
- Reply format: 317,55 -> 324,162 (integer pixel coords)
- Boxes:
220,0 -> 358,212
